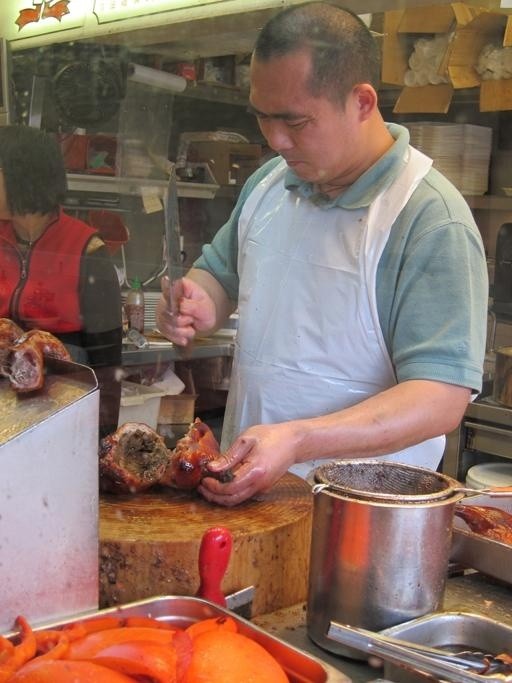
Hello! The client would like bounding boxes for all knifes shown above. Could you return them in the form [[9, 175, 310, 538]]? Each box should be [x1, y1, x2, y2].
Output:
[[163, 164, 194, 360]]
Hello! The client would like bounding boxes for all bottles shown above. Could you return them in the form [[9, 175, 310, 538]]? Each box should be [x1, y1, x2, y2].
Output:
[[121, 139, 160, 177], [127, 276, 144, 335]]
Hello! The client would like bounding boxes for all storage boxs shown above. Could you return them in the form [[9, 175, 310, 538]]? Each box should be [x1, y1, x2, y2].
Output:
[[378, 0, 512, 116]]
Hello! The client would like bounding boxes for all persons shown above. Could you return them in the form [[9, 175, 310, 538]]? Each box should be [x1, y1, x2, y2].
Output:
[[154, 2, 489, 509], [0, 123, 123, 444]]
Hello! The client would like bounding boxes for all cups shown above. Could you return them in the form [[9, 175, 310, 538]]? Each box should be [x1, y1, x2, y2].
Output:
[[306, 465, 467, 664], [403, 34, 450, 86]]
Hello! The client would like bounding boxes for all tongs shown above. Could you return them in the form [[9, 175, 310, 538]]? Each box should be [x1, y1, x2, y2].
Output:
[[323, 614, 511, 682]]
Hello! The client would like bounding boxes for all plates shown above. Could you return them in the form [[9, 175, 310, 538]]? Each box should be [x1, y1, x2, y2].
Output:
[[121, 292, 163, 329], [400, 120, 493, 196], [500, 186, 511, 197]]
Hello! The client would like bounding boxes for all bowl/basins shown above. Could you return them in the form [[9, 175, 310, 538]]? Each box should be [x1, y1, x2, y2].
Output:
[[176, 166, 205, 181]]
